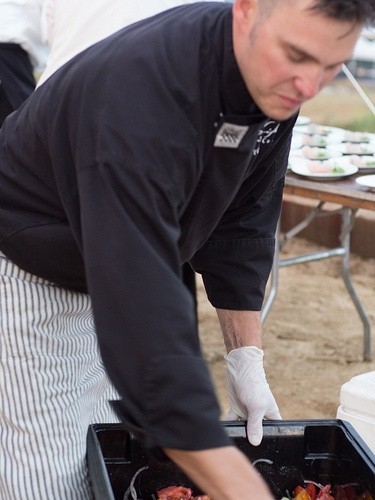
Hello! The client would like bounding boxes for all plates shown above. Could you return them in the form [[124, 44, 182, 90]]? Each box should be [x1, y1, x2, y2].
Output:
[[286, 114, 375, 194]]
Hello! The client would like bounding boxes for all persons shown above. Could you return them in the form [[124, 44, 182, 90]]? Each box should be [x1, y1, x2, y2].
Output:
[[0, 0, 375, 500], [0, 0, 53, 121]]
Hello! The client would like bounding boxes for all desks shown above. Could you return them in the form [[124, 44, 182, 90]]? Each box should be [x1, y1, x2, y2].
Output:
[[261, 171, 375, 363]]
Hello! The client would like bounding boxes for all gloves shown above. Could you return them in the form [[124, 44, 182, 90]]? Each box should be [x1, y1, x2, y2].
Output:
[[222, 346, 282, 446]]
